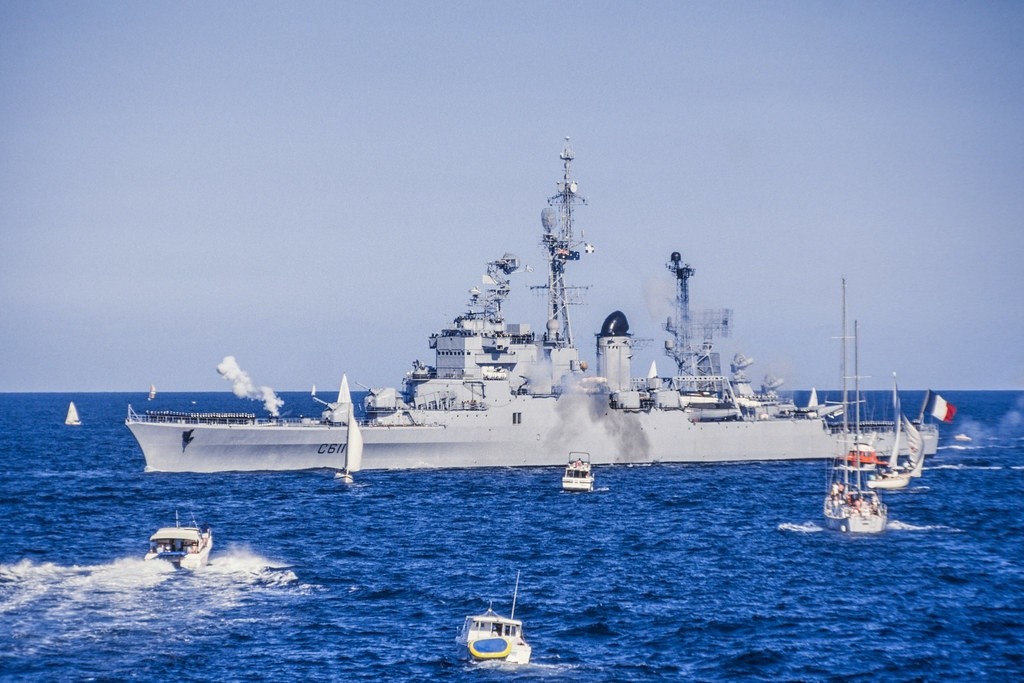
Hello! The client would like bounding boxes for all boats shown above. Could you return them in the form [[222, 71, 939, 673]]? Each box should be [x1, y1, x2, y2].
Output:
[[144, 505, 215, 571], [451, 569, 531, 669], [561, 451, 595, 492]]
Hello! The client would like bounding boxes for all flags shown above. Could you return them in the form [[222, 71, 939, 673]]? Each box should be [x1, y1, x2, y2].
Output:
[[557, 248, 580, 261], [929, 394, 959, 424], [584, 243, 594, 253]]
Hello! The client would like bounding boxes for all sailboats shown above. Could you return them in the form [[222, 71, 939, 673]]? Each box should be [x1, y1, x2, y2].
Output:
[[824, 276, 889, 535], [64, 402, 82, 426], [147, 384, 156, 402], [864, 378, 925, 491]]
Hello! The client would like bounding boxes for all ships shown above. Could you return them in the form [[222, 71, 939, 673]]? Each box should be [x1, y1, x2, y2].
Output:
[[125, 133, 957, 474]]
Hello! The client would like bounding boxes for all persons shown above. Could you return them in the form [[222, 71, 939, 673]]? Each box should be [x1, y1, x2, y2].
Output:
[[144, 410, 273, 425], [877, 468, 901, 480], [509, 329, 548, 344], [828, 483, 885, 517], [579, 458, 584, 466], [158, 541, 198, 554]]
